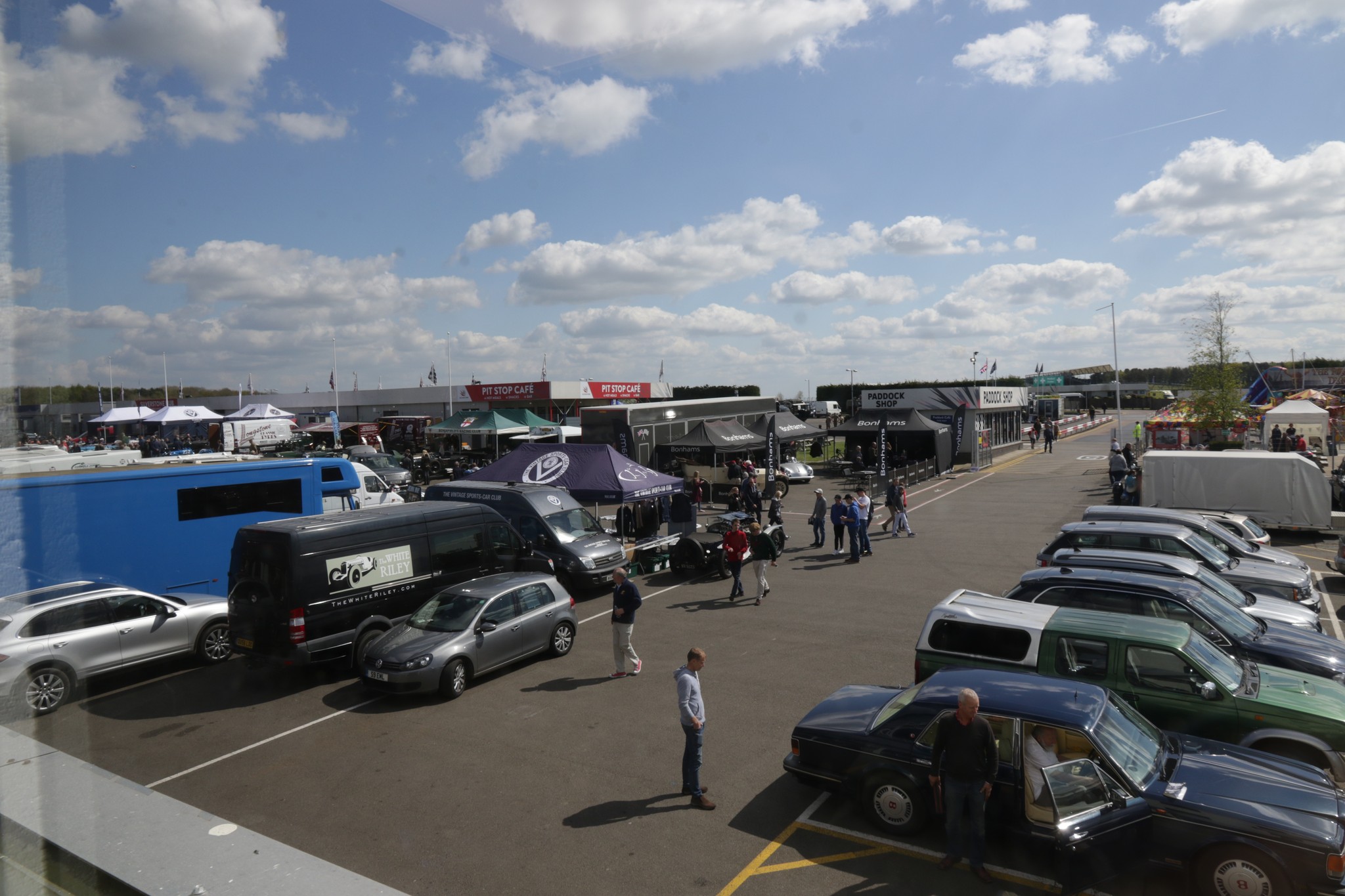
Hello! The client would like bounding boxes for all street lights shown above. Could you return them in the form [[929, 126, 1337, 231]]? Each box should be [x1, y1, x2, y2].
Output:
[[846, 368, 858, 417], [805, 379, 810, 403]]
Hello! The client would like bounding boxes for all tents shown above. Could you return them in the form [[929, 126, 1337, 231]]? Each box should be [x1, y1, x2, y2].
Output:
[[87, 404, 296, 439], [655, 407, 953, 511], [424, 409, 560, 462], [1263, 399, 1330, 455]]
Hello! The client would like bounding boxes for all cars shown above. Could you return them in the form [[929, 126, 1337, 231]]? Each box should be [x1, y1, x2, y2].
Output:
[[72, 427, 114, 443], [805, 401, 816, 419], [999, 504, 1345, 687], [26, 433, 40, 440], [1330, 455, 1345, 508], [781, 665, 1345, 895], [359, 570, 578, 701], [0, 580, 233, 718]]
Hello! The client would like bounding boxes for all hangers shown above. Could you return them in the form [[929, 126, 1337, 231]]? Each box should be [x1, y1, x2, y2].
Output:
[[624, 503, 626, 506]]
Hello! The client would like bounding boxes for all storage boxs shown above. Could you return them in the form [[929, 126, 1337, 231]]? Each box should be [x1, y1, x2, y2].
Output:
[[624, 543, 670, 578]]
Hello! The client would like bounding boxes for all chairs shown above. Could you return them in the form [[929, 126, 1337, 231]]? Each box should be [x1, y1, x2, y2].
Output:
[[1001, 719, 1078, 824], [1151, 539, 1160, 549], [1127, 648, 1192, 692], [1144, 599, 1168, 618], [827, 456, 892, 493]]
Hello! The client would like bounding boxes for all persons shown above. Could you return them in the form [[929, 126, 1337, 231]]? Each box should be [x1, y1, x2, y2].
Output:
[[608, 567, 642, 677], [674, 647, 717, 809], [0, 429, 512, 486], [1134, 421, 1141, 445], [1271, 423, 1306, 452], [1020, 403, 1108, 455], [614, 440, 917, 607], [925, 687, 1000, 884], [1024, 725, 1113, 808], [1109, 438, 1142, 506]]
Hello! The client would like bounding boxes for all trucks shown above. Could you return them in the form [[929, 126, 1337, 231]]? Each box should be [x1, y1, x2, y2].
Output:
[[210, 418, 300, 451]]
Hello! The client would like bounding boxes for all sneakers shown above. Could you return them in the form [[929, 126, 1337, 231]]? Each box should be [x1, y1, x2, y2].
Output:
[[633, 659, 642, 673], [784, 535, 791, 540], [608, 671, 626, 678], [810, 542, 823, 548], [831, 544, 873, 563], [881, 524, 920, 538]]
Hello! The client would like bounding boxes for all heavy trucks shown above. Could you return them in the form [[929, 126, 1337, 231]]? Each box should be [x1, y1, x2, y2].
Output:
[[0, 457, 359, 595]]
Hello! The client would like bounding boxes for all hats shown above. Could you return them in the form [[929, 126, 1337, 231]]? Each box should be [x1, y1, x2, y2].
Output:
[[843, 494, 852, 499], [834, 495, 841, 500], [322, 441, 327, 444], [855, 487, 864, 492], [749, 522, 760, 530], [814, 489, 823, 494], [732, 460, 736, 463]]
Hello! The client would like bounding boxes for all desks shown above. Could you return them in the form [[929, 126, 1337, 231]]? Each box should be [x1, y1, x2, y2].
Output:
[[836, 461, 852, 469], [852, 471, 876, 491], [635, 536, 681, 576], [1311, 454, 1326, 473], [601, 515, 616, 529]]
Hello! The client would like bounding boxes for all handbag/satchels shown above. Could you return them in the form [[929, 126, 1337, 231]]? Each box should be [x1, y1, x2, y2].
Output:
[[700, 492, 703, 497], [774, 511, 784, 524], [885, 501, 888, 506], [750, 466, 759, 477]]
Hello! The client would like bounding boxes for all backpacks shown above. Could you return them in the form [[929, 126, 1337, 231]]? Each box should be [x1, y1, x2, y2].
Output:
[[422, 457, 430, 470]]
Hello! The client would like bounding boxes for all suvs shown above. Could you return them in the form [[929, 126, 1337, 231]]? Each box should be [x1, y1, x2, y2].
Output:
[[913, 587, 1345, 784], [274, 432, 314, 452], [353, 452, 412, 491]]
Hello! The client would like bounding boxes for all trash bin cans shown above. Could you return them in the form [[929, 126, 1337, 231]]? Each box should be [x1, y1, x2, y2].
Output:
[[654, 553, 670, 569], [627, 562, 638, 578], [641, 557, 663, 572]]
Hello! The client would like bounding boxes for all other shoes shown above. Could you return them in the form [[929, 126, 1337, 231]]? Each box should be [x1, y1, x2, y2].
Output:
[[755, 600, 761, 605], [699, 510, 705, 512], [735, 593, 744, 597], [762, 589, 770, 597], [976, 867, 992, 881], [691, 794, 716, 809], [729, 596, 734, 601], [939, 855, 955, 870], [682, 786, 708, 794]]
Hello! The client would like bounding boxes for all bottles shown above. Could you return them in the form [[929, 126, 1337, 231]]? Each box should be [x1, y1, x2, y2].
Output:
[[614, 605, 621, 618], [770, 562, 778, 567]]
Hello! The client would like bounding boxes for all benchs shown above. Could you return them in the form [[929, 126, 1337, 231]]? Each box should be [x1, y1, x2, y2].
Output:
[[1319, 456, 1329, 467]]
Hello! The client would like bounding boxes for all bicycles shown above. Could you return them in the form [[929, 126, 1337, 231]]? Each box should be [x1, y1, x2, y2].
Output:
[[829, 448, 845, 467]]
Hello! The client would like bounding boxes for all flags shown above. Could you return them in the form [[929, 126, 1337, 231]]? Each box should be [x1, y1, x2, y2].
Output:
[[178, 382, 183, 398], [248, 374, 255, 396], [119, 384, 124, 401], [542, 358, 546, 382], [304, 364, 475, 393], [979, 361, 1043, 376]]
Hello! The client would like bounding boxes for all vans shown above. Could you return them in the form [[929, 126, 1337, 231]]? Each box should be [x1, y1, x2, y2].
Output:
[[322, 460, 405, 514], [1150, 390, 1175, 399], [227, 499, 556, 679], [424, 479, 632, 591], [1058, 393, 1085, 408], [816, 401, 842, 418]]
